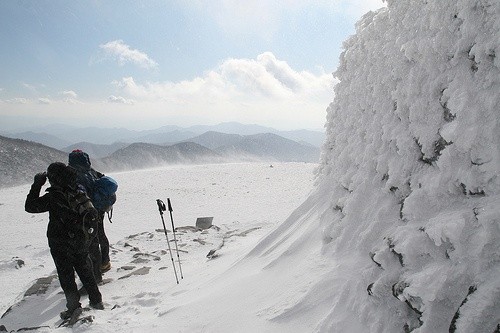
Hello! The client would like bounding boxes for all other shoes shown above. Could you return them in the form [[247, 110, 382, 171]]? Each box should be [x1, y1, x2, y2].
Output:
[[79, 285, 89, 296], [59, 308, 81, 320], [93, 274, 101, 283], [99, 262, 111, 273], [88, 302, 106, 310]]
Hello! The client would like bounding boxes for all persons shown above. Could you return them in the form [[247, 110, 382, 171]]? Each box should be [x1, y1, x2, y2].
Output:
[[68, 149, 111, 294], [25, 162, 104, 321]]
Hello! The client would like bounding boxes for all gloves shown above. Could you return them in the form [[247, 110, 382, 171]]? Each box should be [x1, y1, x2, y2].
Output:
[[34, 171, 48, 186]]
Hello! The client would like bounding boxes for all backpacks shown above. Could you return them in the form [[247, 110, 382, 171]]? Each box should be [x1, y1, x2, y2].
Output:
[[50, 185, 99, 250], [86, 169, 118, 224]]
